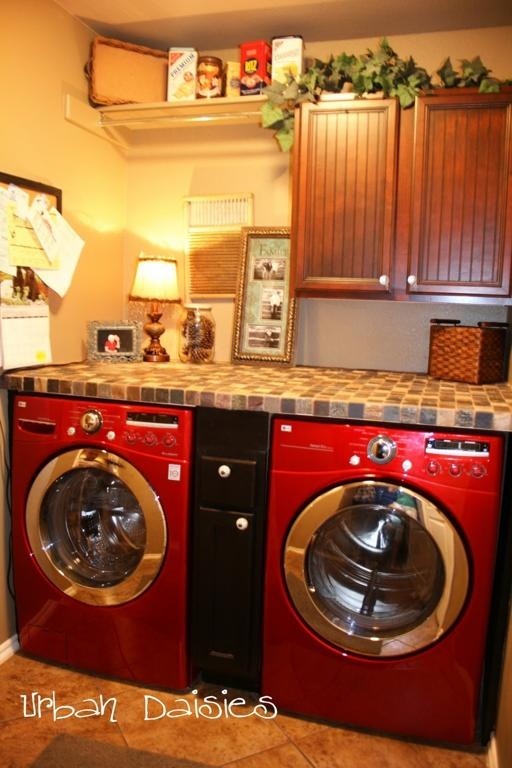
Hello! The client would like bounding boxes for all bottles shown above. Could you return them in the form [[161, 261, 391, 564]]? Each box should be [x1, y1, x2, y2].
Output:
[[177, 303, 216, 363]]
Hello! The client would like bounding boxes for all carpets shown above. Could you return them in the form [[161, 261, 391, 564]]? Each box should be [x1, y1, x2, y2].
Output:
[[29, 733, 216, 768]]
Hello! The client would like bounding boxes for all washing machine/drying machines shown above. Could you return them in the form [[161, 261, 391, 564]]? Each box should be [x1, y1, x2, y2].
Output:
[[262, 415, 505, 751], [8, 393, 197, 696]]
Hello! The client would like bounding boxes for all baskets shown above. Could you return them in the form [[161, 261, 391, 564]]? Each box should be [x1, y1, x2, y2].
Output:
[[428, 323, 506, 387]]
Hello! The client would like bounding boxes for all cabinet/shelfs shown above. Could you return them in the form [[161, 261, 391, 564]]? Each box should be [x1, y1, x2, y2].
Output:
[[288, 79, 512, 305]]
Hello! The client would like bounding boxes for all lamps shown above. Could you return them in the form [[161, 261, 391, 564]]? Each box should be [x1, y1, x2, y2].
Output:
[[128, 256, 181, 363]]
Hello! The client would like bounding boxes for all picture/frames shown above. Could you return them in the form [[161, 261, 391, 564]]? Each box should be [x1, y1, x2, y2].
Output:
[[231, 225, 298, 366], [87, 320, 143, 364]]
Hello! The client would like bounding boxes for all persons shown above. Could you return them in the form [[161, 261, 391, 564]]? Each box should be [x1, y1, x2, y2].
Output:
[[264, 330, 280, 346], [268, 260, 280, 279], [271, 291, 280, 318], [104, 333, 121, 353], [259, 260, 270, 278]]
